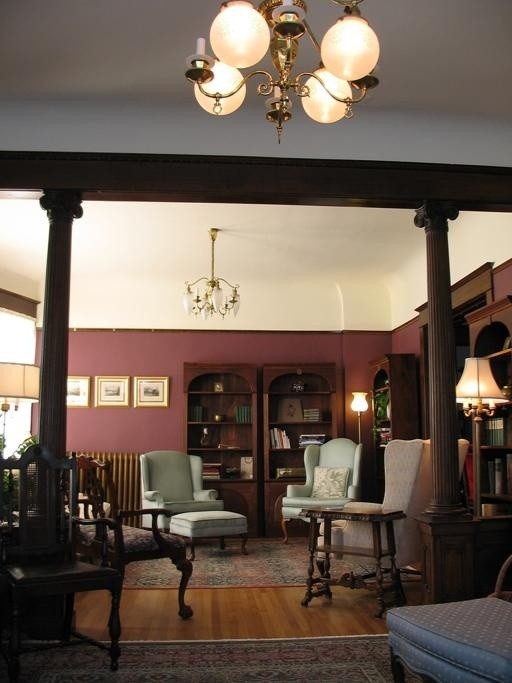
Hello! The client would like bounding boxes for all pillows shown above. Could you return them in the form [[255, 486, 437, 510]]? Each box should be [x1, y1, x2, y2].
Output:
[[311, 464, 350, 501]]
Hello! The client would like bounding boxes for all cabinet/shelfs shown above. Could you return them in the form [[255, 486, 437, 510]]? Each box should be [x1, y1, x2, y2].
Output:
[[461, 289, 511, 521], [180, 359, 261, 538], [254, 362, 340, 538], [366, 351, 422, 502]]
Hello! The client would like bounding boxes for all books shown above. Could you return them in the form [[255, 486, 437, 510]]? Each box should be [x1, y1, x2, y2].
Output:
[[189, 407, 203, 422], [235, 406, 252, 423], [303, 409, 322, 422], [485, 417, 512, 495], [270, 428, 290, 449], [203, 463, 223, 479]]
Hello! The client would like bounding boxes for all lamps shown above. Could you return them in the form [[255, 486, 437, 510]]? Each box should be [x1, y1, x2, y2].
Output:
[[451, 354, 508, 521], [349, 389, 369, 442], [183, 0, 387, 144], [0, 360, 41, 454], [183, 224, 243, 320]]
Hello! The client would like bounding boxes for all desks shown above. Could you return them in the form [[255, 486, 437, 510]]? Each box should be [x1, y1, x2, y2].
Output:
[[295, 503, 406, 624]]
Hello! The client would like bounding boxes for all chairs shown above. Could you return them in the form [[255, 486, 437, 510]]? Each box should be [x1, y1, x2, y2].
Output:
[[62, 449, 200, 625], [374, 543, 512, 683], [311, 435, 467, 583], [1, 443, 127, 683], [279, 434, 370, 543], [138, 445, 226, 556]]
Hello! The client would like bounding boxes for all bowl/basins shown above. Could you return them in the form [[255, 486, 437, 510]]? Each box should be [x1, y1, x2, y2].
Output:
[[213, 414, 226, 422]]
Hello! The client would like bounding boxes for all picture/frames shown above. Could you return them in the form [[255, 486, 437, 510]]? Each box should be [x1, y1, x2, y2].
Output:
[[131, 373, 170, 408], [66, 372, 93, 409], [93, 374, 132, 409]]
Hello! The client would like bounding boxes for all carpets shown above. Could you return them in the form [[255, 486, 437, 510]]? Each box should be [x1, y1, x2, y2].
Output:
[[76, 529, 423, 592], [0, 624, 435, 681]]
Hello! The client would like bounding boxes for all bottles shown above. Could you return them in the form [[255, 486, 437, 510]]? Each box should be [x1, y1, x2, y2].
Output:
[[501, 386, 512, 409], [200, 428, 210, 448]]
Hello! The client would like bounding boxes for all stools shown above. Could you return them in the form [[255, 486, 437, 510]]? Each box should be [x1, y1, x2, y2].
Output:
[[165, 508, 250, 560]]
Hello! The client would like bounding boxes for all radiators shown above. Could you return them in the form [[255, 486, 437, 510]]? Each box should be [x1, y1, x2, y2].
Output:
[[62, 446, 146, 530]]
